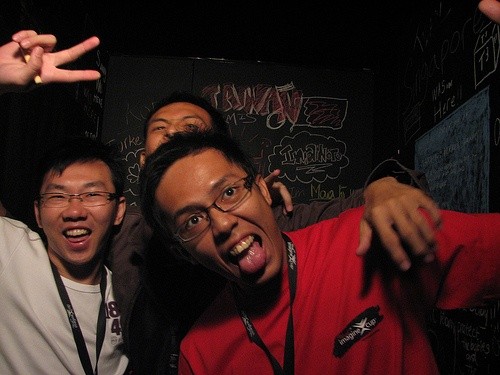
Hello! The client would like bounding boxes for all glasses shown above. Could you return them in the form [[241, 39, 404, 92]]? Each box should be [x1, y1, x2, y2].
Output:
[[170, 172, 257, 243], [37, 190, 120, 208]]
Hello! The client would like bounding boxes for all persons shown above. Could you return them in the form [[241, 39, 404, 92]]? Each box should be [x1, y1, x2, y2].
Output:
[[104, 91, 443, 375], [137, 1, 500, 375], [0, 30, 129, 375]]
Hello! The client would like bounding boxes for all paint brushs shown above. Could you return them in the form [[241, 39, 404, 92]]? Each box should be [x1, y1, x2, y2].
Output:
[[17, 39, 42, 85]]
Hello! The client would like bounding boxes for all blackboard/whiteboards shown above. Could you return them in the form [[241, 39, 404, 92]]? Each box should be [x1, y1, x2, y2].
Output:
[[78, 1, 500, 375]]
